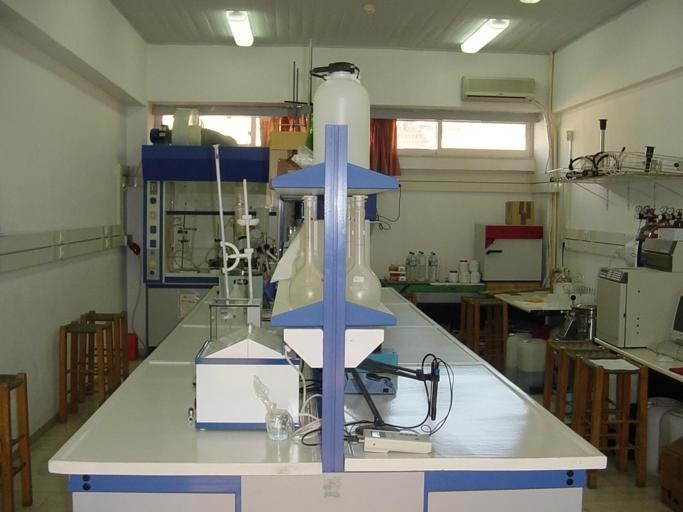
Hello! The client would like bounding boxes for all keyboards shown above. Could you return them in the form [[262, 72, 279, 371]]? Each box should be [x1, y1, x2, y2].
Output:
[[647, 340, 683, 361]]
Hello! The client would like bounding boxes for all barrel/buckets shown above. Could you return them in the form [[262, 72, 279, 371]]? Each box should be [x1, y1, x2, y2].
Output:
[[660, 408, 683, 469], [517, 339, 547, 388], [570, 304, 597, 340], [635, 397, 681, 475], [505, 333, 532, 383]]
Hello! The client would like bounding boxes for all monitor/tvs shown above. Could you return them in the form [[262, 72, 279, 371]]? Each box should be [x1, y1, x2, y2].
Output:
[[671, 295, 683, 340]]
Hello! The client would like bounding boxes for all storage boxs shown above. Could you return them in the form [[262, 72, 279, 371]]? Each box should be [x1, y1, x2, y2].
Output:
[[657, 432, 682, 512]]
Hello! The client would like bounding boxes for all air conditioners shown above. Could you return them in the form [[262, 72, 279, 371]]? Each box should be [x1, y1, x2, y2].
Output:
[[458, 74, 538, 105]]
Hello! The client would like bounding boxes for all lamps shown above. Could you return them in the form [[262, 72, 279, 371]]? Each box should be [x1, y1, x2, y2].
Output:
[[454, 15, 513, 56], [221, 6, 255, 51]]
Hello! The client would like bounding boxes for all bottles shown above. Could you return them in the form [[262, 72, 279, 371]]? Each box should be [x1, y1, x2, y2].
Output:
[[265, 408, 294, 440], [289, 195, 381, 309], [235, 187, 246, 248], [549, 266, 596, 306], [406, 250, 482, 283], [230, 284, 241, 309]]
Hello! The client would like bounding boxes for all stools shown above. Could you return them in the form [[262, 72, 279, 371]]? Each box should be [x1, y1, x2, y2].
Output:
[[457, 292, 505, 367], [562, 347, 621, 470], [79, 305, 130, 401], [572, 356, 649, 491], [54, 320, 117, 423], [542, 333, 607, 425], [0, 368, 38, 512]]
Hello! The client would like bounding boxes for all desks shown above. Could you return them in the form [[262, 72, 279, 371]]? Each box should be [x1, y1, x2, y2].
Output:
[[48, 268, 611, 512], [378, 280, 486, 306], [493, 291, 595, 316], [592, 334, 682, 391]]
